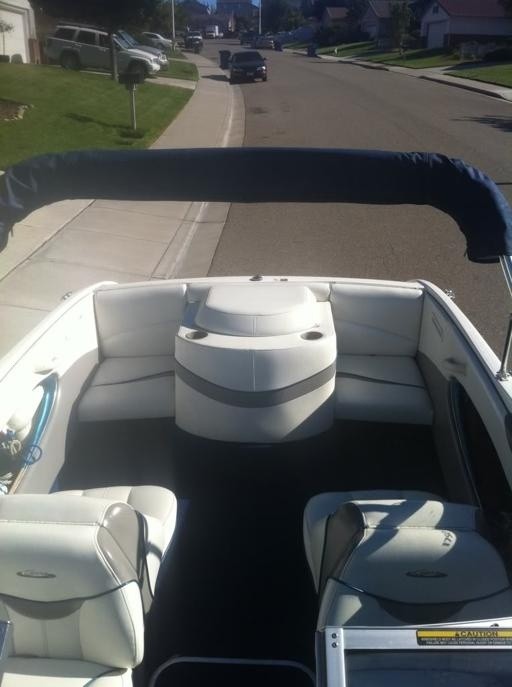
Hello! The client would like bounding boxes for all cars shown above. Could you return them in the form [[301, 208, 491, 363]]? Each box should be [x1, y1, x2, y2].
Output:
[[43, 22, 174, 79], [228, 51, 268, 82], [186, 31, 202, 46], [241, 32, 273, 49]]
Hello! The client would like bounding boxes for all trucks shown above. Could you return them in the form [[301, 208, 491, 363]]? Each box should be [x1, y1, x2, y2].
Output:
[[206, 25, 224, 39]]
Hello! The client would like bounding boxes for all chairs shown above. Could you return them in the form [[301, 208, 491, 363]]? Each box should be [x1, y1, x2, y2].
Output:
[[330, 284, 436, 438], [303, 491, 511, 626], [1, 483, 183, 687], [74, 283, 186, 439]]
